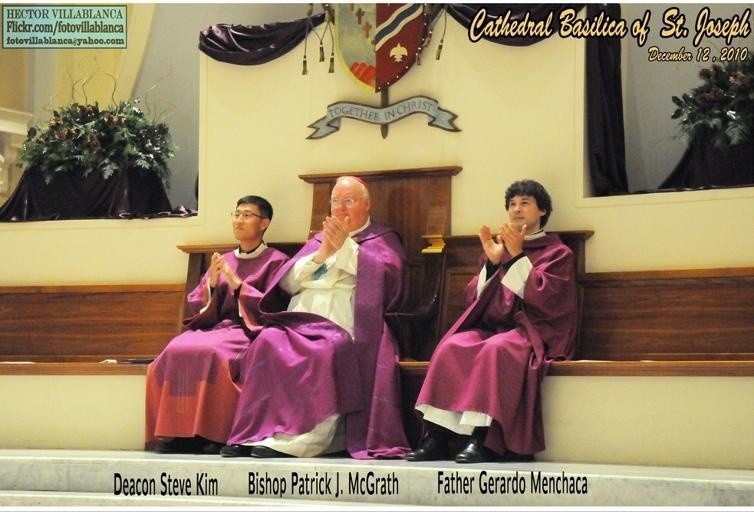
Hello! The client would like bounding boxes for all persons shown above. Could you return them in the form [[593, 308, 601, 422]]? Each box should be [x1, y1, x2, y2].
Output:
[[216, 174, 413, 460], [405, 179, 585, 464], [145, 194, 291, 454]]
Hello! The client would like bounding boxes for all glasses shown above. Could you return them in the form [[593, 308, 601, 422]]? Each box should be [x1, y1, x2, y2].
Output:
[[329, 197, 354, 209], [230, 210, 266, 219]]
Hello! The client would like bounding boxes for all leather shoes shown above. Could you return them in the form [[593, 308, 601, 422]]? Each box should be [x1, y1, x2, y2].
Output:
[[407, 434, 444, 460], [455, 438, 490, 463], [221, 443, 286, 455]]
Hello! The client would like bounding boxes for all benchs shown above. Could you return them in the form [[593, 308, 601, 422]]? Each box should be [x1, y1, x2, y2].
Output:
[[0, 167, 754, 377]]
